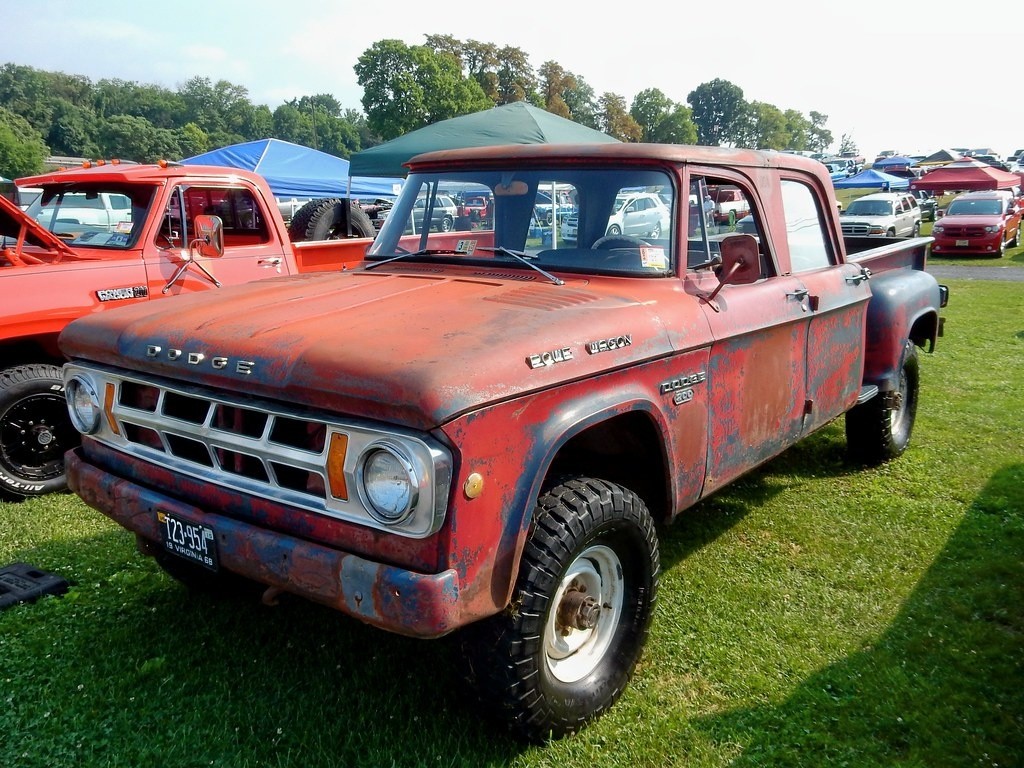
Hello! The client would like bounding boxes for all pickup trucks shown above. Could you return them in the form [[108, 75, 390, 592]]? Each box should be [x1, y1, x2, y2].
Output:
[[0, 159, 495, 500], [58, 142, 948, 740]]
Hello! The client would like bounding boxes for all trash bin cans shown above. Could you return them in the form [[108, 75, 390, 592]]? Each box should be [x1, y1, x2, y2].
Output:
[[542, 229, 552, 246]]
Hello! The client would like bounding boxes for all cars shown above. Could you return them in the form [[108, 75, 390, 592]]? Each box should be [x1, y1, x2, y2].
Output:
[[17, 188, 134, 234], [758, 148, 900, 177], [405, 192, 560, 233], [689, 189, 938, 238], [930, 191, 1021, 258], [561, 193, 670, 244]]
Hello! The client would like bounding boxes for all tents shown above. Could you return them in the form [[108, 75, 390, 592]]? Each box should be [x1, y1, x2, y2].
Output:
[[169, 139, 416, 248], [347, 102, 624, 250], [832, 149, 1022, 193]]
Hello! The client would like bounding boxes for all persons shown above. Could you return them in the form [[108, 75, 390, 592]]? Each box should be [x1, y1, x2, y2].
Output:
[[704, 195, 715, 227]]
